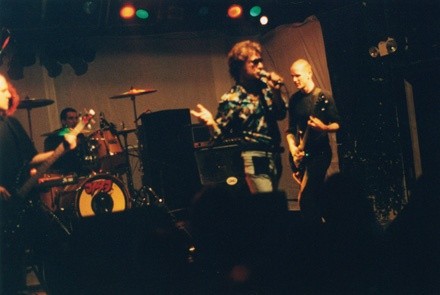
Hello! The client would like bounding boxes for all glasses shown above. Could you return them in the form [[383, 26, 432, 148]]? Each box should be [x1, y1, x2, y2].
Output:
[[251, 58, 263, 64]]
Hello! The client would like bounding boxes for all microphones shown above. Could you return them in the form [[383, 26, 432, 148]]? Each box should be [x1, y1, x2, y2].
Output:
[[259, 70, 285, 86], [100, 113, 104, 129]]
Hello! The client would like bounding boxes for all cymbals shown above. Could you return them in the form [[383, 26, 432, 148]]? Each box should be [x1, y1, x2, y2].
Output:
[[117, 127, 136, 135], [109, 86, 159, 100], [37, 127, 95, 138], [8, 96, 56, 110]]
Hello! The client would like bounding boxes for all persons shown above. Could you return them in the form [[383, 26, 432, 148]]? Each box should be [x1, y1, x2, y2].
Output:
[[189, 40, 288, 194], [0, 75, 78, 295], [285, 58, 345, 211], [44, 108, 100, 177]]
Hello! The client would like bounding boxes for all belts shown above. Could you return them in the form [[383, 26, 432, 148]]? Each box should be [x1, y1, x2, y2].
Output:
[[240, 145, 284, 153]]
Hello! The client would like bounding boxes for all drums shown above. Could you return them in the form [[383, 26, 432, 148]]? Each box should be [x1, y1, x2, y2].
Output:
[[55, 173, 131, 216], [34, 173, 62, 213], [85, 126, 128, 170]]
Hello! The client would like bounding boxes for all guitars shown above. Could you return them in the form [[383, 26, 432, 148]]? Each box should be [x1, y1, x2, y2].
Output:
[[288, 91, 330, 177], [1, 106, 97, 241]]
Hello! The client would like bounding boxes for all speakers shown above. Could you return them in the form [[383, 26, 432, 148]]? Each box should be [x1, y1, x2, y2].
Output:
[[140, 109, 238, 217]]
